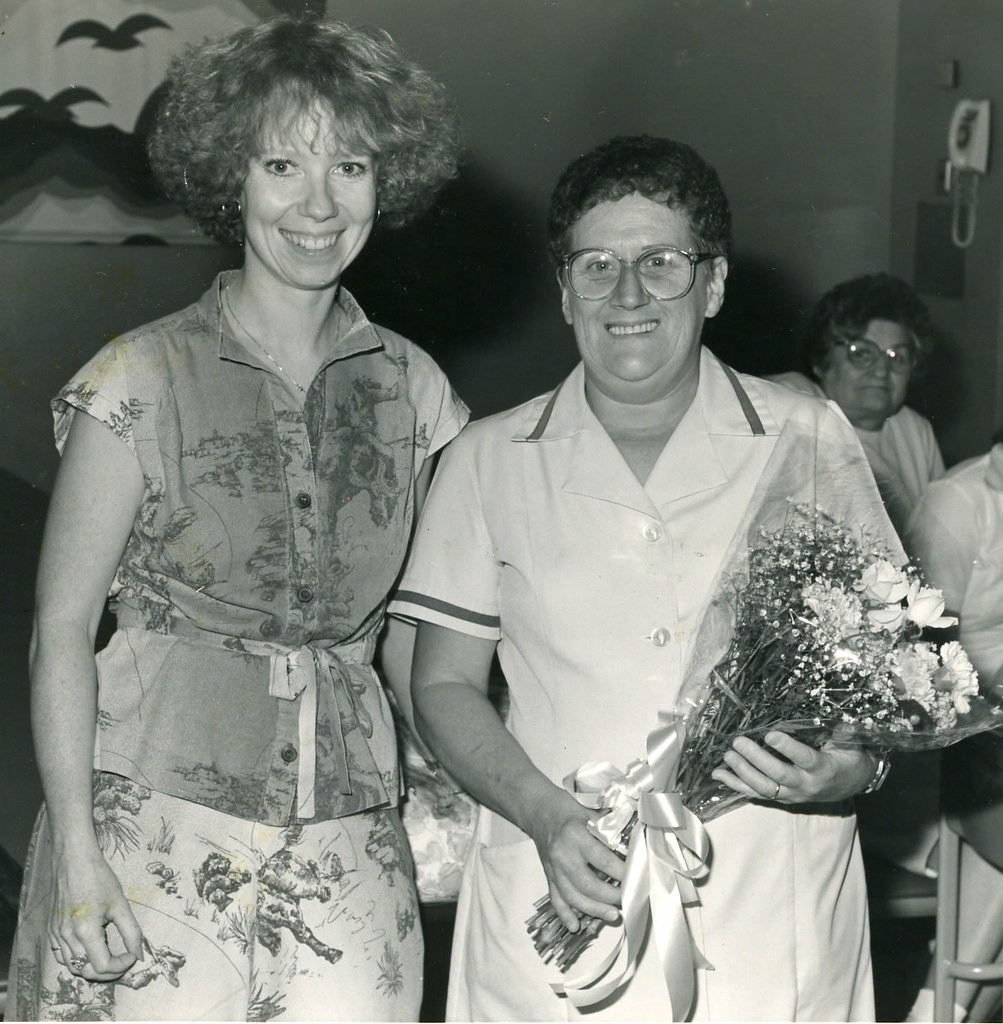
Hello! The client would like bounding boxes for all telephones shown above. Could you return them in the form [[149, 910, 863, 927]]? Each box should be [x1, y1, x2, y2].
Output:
[[948, 99, 991, 174]]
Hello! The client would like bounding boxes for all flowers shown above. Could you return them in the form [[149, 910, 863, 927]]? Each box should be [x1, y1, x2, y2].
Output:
[[528, 478, 1003, 969]]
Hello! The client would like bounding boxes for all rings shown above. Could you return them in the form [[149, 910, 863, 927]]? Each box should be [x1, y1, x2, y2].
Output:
[[50, 946, 61, 950], [70, 953, 89, 968], [769, 783, 779, 800]]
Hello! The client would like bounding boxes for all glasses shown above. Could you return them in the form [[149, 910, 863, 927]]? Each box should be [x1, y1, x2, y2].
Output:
[[829, 337, 921, 374], [558, 247, 719, 301]]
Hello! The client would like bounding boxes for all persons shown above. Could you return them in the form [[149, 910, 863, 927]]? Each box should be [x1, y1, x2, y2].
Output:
[[383, 130, 913, 1022], [3, 19, 471, 1024], [808, 271, 944, 553], [908, 422, 1001, 1020]]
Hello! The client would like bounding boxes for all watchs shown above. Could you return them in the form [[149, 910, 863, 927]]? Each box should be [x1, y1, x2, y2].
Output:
[[866, 760, 890, 794]]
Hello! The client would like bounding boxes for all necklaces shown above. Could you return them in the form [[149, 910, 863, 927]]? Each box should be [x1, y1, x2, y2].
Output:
[[223, 281, 342, 395]]
